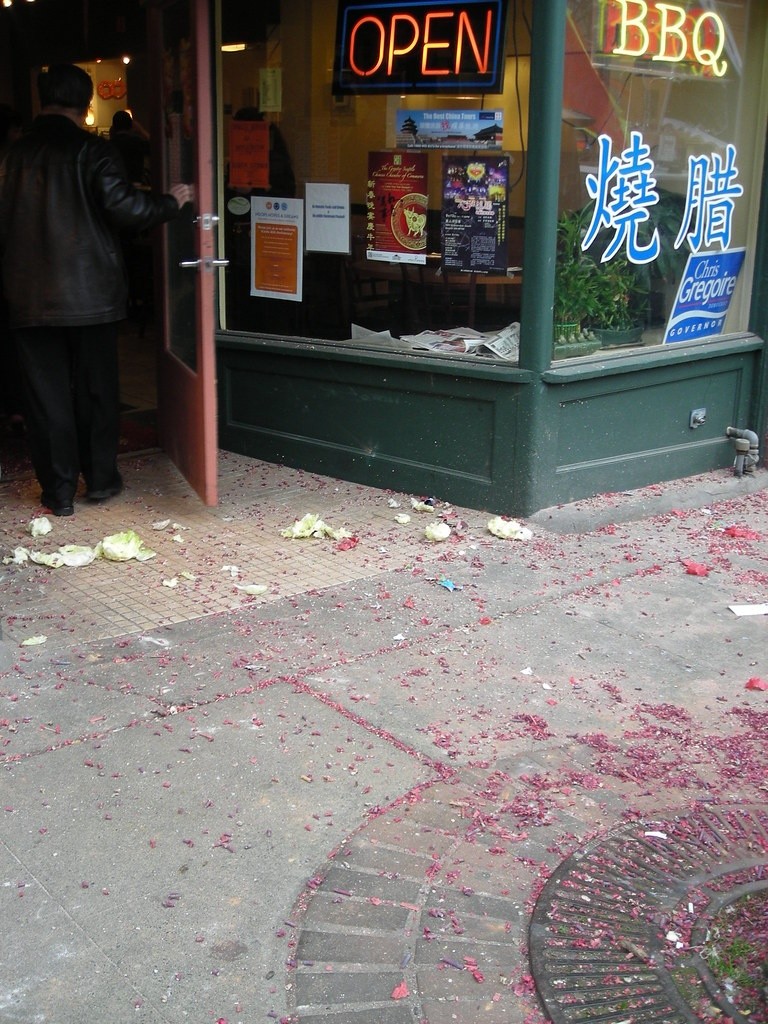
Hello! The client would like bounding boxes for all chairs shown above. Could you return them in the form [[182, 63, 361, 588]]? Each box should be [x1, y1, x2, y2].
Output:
[[400, 263, 481, 334]]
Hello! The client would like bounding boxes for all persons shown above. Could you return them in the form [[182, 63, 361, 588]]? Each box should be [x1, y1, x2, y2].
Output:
[[0, 60, 191, 516], [226, 106, 296, 222]]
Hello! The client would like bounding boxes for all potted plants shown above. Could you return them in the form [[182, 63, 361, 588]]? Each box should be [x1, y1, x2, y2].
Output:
[[555, 200, 650, 360]]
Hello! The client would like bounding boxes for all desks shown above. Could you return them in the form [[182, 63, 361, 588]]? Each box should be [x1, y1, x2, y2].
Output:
[[354, 261, 522, 319]]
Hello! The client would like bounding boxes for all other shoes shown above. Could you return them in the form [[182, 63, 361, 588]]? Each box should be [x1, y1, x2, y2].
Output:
[[87, 471, 123, 499], [41, 492, 74, 516], [6, 421, 31, 437]]
